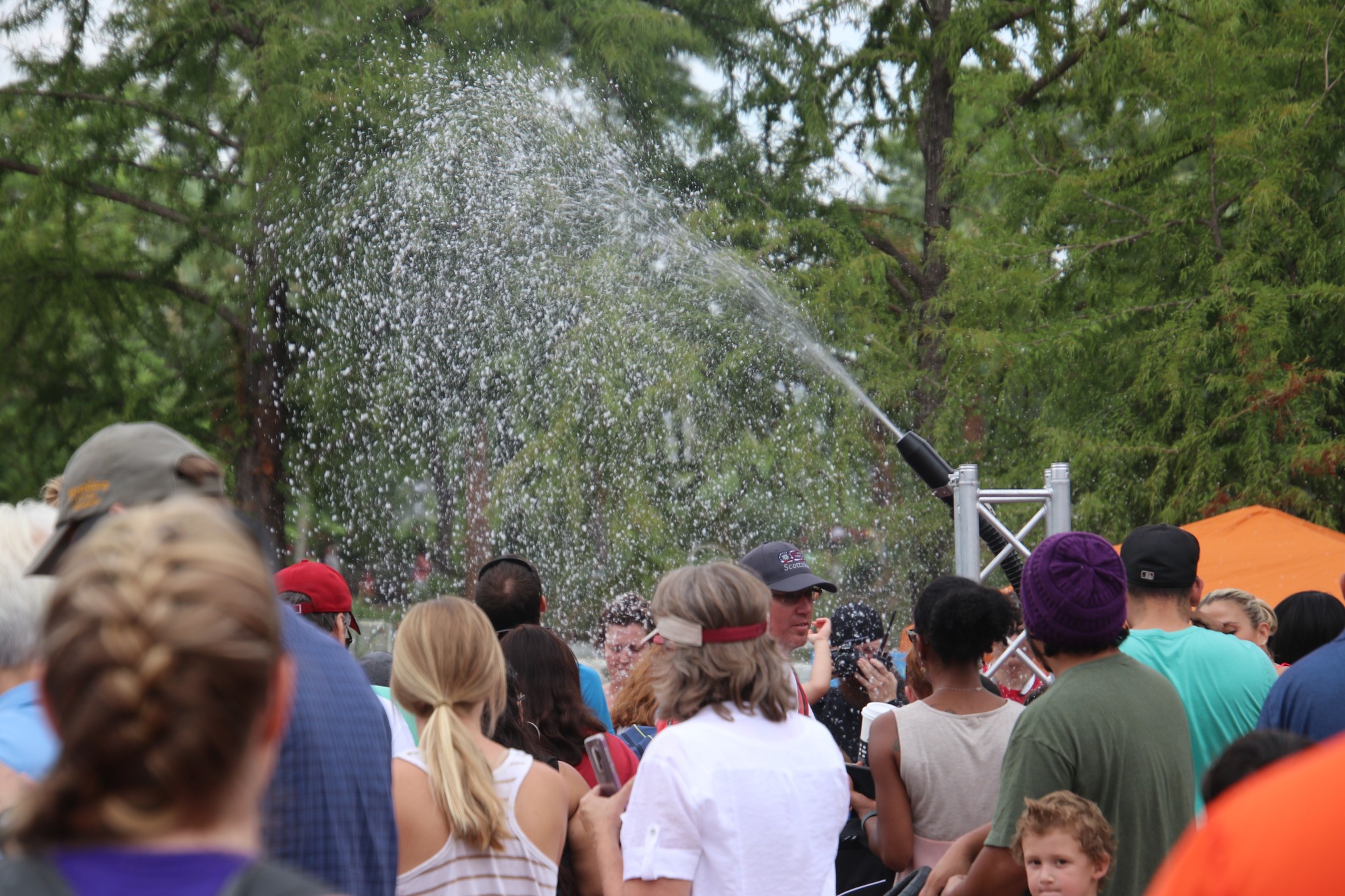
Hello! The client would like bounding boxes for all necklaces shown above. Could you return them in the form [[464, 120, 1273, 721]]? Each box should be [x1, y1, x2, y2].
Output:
[[931, 686, 986, 692]]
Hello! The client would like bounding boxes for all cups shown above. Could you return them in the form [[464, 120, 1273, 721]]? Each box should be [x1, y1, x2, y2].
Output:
[[858, 701, 898, 767]]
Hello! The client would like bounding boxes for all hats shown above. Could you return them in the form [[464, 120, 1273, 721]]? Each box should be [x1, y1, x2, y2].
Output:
[[1018, 530, 1129, 637], [831, 602, 886, 646], [735, 541, 838, 595], [266, 560, 365, 637], [1265, 590, 1345, 665], [20, 420, 225, 575], [1117, 521, 1201, 585]]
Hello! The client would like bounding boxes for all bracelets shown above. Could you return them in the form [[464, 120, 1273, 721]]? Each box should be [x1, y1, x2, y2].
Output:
[[860, 810, 878, 832], [887, 699, 899, 708]]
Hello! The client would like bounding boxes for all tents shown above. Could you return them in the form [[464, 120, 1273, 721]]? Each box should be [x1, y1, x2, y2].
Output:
[[899, 504, 1345, 654]]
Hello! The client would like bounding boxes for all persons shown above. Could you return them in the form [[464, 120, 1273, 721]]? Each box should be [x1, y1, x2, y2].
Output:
[[0, 423, 1345, 896]]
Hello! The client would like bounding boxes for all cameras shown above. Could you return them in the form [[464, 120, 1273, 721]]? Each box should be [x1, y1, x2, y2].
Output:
[[830, 645, 865, 677]]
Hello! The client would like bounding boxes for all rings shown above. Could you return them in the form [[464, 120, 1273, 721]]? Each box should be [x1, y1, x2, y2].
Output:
[[869, 676, 874, 682]]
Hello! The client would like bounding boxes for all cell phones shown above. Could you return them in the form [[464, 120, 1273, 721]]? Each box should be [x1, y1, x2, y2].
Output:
[[585, 736, 627, 811]]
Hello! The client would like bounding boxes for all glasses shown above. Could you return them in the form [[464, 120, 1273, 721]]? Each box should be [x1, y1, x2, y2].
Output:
[[771, 584, 823, 606]]
